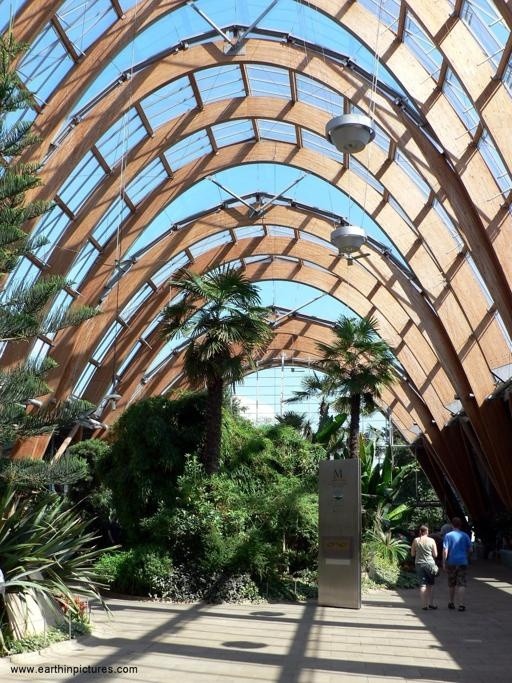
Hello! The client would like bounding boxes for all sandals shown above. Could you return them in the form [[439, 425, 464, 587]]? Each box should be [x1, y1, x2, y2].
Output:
[[448, 602, 455, 609], [458, 605, 466, 612]]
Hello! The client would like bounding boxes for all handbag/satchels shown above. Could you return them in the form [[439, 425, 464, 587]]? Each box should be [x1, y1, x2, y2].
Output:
[[431, 565, 439, 576]]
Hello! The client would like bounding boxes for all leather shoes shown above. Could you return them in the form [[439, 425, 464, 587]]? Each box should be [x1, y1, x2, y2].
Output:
[[422, 607, 428, 610], [428, 605, 437, 609]]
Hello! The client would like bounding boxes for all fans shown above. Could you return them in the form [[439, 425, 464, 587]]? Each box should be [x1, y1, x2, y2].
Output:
[[329, 215, 370, 266]]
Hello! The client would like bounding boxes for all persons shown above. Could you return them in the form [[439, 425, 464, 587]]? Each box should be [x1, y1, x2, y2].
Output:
[[441, 520, 452, 540], [411, 524, 439, 610], [443, 518, 473, 611]]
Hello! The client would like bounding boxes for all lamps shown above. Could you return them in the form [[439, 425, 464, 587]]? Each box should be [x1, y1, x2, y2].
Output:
[[104, 102, 126, 411], [316, 136, 367, 253], [298, 0, 385, 155]]
